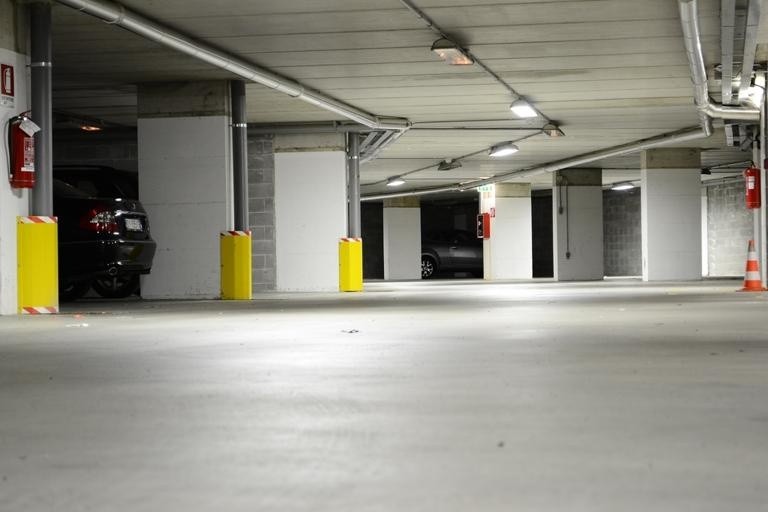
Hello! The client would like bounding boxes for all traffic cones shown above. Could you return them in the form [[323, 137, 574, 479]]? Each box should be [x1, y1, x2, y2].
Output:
[[736, 239, 768, 292]]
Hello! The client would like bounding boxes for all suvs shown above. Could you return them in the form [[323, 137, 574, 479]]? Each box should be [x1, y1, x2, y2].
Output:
[[53, 162, 157, 305]]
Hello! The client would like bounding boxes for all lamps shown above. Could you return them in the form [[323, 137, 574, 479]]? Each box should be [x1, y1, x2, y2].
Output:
[[385, 123, 564, 189], [431, 38, 539, 120]]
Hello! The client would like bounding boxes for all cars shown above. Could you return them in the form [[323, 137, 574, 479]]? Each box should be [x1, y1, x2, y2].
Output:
[[370, 227, 483, 279]]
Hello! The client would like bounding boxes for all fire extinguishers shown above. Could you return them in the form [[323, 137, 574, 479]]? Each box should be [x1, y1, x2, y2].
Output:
[[8, 110, 36, 188], [744, 160, 760, 208]]
